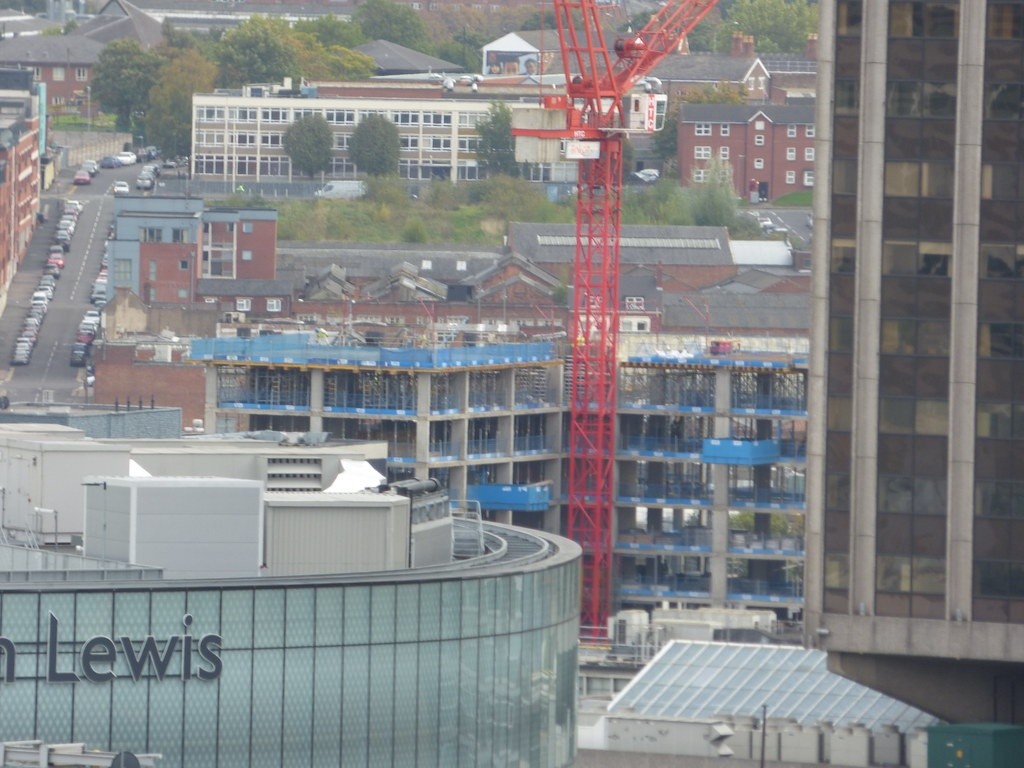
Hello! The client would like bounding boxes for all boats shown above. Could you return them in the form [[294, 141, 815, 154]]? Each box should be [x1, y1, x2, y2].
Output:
[[11, 201, 83, 366], [71, 219, 115, 369]]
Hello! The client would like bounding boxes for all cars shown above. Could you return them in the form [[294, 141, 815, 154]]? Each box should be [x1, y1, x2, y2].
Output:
[[163, 154, 188, 169], [743, 208, 813, 251], [134, 145, 162, 162], [73, 171, 91, 185], [136, 164, 160, 189], [114, 181, 130, 197], [81, 151, 137, 176]]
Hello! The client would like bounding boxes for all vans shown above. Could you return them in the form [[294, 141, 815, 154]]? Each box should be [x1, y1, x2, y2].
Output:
[[314, 180, 365, 199]]
